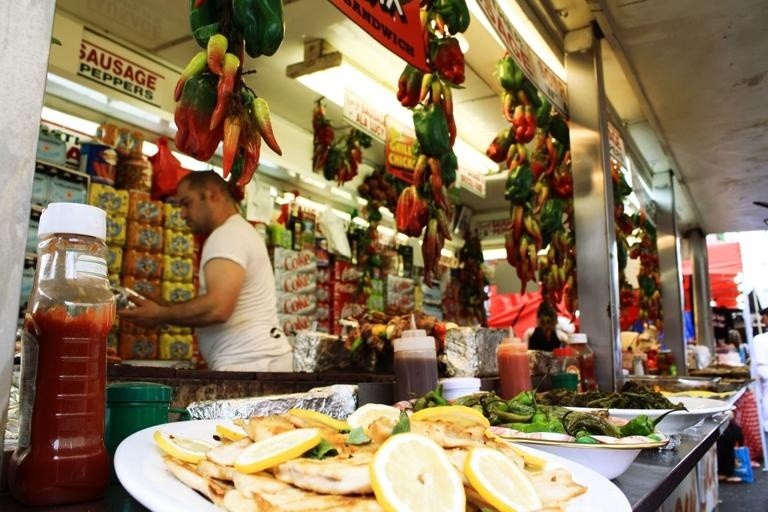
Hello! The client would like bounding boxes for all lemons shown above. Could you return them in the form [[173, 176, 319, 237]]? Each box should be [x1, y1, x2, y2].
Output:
[[153, 402, 587, 512]]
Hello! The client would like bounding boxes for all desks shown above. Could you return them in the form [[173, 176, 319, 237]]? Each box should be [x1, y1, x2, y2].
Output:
[[109, 404, 730, 512]]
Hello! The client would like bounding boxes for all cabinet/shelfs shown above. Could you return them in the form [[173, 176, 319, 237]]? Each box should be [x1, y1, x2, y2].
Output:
[[13, 124, 441, 382]]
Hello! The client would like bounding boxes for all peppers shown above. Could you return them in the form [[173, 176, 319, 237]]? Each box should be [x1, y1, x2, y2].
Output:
[[174, 0, 286, 186], [312, 0, 664, 336], [404, 366, 690, 443]]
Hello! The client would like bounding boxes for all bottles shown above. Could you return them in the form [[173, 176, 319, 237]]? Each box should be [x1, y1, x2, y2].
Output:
[[567, 333, 599, 393], [271, 201, 306, 252], [34, 124, 87, 187], [631, 344, 677, 377], [496, 325, 533, 399], [389, 314, 439, 408], [7, 201, 118, 509], [549, 345, 584, 394], [81, 118, 154, 196]]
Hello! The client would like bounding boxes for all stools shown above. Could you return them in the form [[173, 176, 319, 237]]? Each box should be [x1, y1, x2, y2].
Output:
[[734, 446, 755, 482]]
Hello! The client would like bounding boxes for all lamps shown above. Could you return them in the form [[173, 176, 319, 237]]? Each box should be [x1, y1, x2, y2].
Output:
[[284, 39, 501, 181], [46, 69, 287, 176]]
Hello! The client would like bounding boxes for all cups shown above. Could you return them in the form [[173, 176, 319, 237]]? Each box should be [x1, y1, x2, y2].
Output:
[[103, 379, 193, 458], [439, 376, 483, 403], [548, 369, 590, 398]]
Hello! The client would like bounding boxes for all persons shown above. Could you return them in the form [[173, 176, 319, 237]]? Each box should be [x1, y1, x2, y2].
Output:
[[528, 300, 561, 351], [116, 170, 294, 373], [713, 307, 768, 485], [655, 315, 666, 350]]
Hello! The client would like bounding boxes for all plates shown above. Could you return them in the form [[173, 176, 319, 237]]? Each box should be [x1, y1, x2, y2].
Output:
[[660, 367, 753, 399], [555, 390, 736, 449], [111, 415, 634, 512], [394, 393, 667, 480]]
